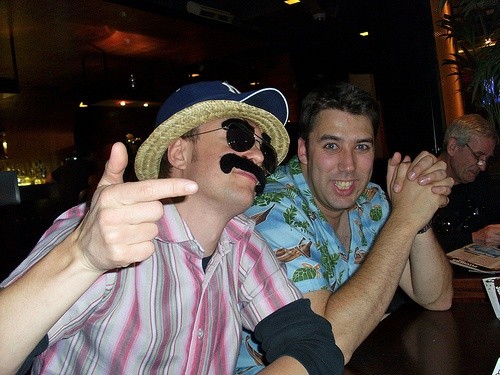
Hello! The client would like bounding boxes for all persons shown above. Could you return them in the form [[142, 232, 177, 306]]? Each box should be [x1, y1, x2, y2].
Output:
[[233, 81, 454, 375], [431, 113, 500, 254], [0, 81, 345, 375]]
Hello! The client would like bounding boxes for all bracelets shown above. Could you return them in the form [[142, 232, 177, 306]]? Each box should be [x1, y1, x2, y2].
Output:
[[416, 219, 432, 234]]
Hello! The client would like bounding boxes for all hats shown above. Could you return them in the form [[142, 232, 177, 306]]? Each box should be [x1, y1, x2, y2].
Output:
[[157, 80, 289, 126], [134, 98, 291, 181]]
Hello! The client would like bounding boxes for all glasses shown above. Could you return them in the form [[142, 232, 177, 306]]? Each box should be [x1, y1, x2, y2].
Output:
[[450, 137, 496, 165], [181, 122, 277, 177]]
